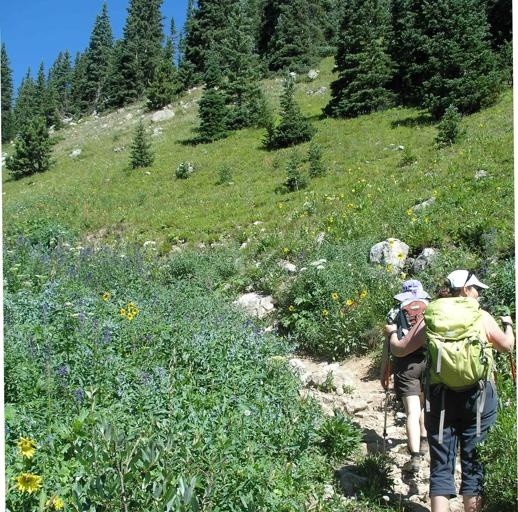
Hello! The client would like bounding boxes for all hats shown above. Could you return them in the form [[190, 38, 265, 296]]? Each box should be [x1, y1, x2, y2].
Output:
[[447, 268, 489, 291], [393, 280, 432, 302]]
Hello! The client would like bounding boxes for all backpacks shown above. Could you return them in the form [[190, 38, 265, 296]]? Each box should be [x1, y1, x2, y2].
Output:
[[397, 297, 428, 339], [424, 297, 493, 389]]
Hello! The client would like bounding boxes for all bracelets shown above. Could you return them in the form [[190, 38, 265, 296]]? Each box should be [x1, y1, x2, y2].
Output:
[[388, 329, 398, 337], [502, 321, 512, 327]]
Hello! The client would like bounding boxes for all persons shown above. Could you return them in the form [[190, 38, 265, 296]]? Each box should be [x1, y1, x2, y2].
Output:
[[384, 269, 517, 511], [380, 278, 433, 475]]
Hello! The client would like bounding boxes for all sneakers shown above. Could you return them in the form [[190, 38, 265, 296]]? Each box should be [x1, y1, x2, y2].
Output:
[[419, 437, 429, 455], [400, 453, 422, 473]]
